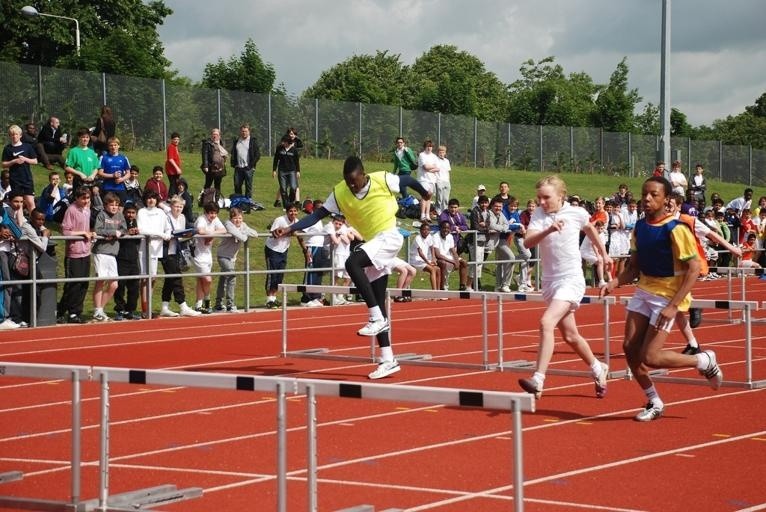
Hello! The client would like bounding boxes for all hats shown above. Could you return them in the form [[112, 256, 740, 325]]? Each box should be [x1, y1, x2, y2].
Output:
[[477, 184, 486, 191]]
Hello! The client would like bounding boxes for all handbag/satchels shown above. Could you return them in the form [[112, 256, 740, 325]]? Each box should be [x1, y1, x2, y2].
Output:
[[454, 236, 466, 254], [176, 248, 190, 274], [7, 248, 30, 278]]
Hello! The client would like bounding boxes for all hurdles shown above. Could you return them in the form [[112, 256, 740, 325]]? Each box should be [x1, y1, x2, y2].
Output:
[[618, 294, 766, 391], [1, 360, 178, 512], [278, 283, 417, 363], [496, 291, 630, 379], [389, 288, 529, 372], [294, 377, 538, 512], [89, 365, 297, 512], [693, 269, 766, 325]]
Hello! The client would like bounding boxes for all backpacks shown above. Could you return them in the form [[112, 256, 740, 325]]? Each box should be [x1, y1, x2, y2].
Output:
[[198, 187, 227, 209]]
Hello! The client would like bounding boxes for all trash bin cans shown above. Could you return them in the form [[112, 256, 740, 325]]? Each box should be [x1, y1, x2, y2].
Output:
[[34, 240, 58, 327]]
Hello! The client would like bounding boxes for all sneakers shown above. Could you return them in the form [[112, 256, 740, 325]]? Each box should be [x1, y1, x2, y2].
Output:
[[264, 294, 353, 306], [599, 280, 608, 288], [493, 274, 534, 292], [636, 400, 664, 423], [592, 360, 609, 398], [680, 344, 701, 356], [690, 307, 701, 328], [54, 302, 151, 325], [158, 299, 239, 318], [518, 376, 542, 399], [0, 317, 29, 330], [393, 294, 412, 302], [366, 359, 400, 380], [701, 349, 723, 391], [355, 318, 391, 336], [707, 271, 721, 281]]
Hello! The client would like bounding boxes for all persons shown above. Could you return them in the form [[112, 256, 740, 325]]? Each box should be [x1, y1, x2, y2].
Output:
[[389, 137, 765, 304], [517, 175, 613, 398], [263, 200, 366, 310], [600, 177, 723, 422], [1, 106, 261, 330], [273, 127, 304, 209], [668, 192, 742, 355], [272, 157, 432, 380]]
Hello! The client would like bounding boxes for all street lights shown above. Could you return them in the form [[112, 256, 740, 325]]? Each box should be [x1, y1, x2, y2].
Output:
[[21, 4, 82, 59]]
